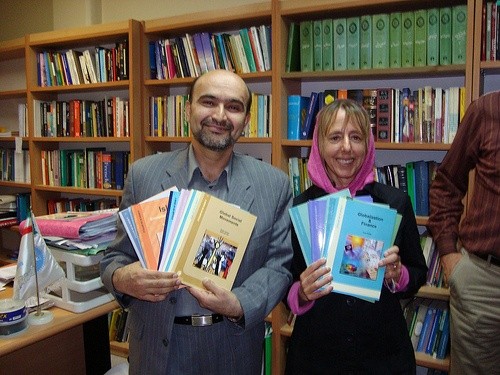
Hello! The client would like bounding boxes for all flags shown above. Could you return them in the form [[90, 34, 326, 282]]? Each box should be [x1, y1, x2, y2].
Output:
[[13, 209, 66, 305]]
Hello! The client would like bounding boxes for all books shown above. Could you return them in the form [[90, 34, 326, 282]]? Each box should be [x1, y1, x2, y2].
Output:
[[287, 4, 467, 72], [417, 230, 450, 290], [287, 147, 314, 198], [244, 92, 273, 138], [47, 198, 117, 215], [403, 295, 450, 360], [263, 324, 273, 375], [149, 93, 190, 138], [482, 0, 500, 63], [0, 141, 31, 183], [107, 307, 132, 345], [289, 188, 402, 303], [374, 161, 436, 218], [117, 186, 256, 295], [149, 25, 271, 80], [0, 193, 28, 229], [37, 39, 128, 87], [40, 146, 129, 192], [288, 86, 466, 145], [0, 102, 28, 137], [37, 93, 129, 138]]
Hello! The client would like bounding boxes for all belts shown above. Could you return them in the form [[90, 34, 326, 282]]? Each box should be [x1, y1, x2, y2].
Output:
[[173, 312, 223, 327], [471, 251, 500, 269]]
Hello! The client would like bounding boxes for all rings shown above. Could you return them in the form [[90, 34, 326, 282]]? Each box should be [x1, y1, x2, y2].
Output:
[[393, 265, 397, 271]]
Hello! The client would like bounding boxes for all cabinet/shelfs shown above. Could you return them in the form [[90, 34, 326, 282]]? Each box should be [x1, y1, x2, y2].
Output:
[[0, 0, 500, 375]]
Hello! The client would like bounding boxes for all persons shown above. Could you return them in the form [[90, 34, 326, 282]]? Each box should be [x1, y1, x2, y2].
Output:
[[279, 99, 428, 375], [94, 72, 297, 375], [429, 90, 500, 375]]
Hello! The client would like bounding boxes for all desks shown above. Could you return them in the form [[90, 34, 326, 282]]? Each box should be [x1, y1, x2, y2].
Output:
[[0, 262, 120, 375]]
